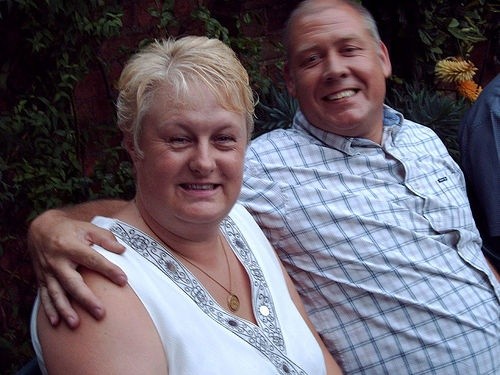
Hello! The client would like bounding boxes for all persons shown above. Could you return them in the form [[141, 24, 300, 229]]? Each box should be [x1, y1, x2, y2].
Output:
[[28, 0, 500, 375], [31, 36, 345, 375]]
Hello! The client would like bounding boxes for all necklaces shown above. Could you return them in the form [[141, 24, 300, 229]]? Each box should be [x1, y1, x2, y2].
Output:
[[146, 222, 241, 312]]
